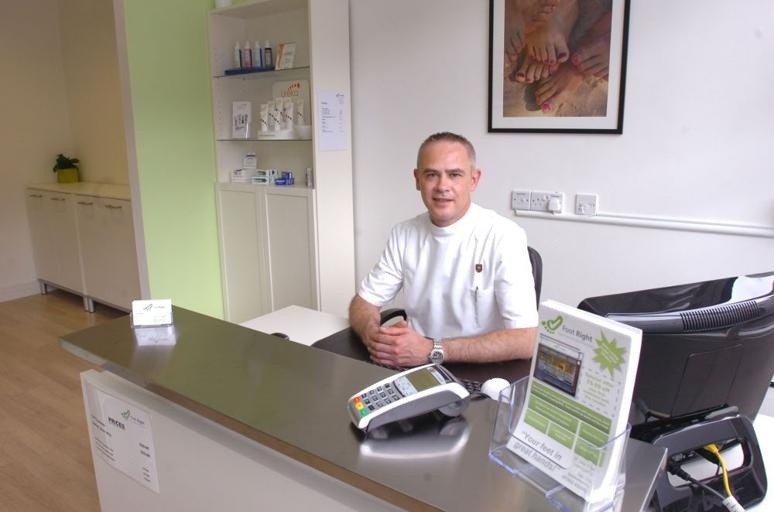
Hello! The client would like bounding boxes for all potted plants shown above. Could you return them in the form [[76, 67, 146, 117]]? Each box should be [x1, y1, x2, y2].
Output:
[[53, 152, 80, 182]]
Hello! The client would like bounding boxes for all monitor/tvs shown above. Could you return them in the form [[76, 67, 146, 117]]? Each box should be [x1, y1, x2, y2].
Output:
[[577, 271, 774, 511]]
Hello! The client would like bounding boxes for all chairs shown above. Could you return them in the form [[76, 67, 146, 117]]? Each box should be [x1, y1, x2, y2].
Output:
[[575, 270, 774, 454], [526, 248, 542, 308]]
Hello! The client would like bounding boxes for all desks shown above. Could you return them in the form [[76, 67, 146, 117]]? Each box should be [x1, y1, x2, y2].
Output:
[[58, 304, 672, 510]]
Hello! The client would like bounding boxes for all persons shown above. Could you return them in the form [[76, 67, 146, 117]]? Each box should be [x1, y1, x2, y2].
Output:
[[504, 0, 612, 115], [349, 131, 539, 368]]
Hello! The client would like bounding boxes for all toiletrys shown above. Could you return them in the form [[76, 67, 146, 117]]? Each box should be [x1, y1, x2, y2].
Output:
[[232, 38, 272, 69], [259, 97, 304, 134]]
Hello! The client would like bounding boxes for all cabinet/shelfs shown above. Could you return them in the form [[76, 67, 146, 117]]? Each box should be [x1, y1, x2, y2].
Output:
[[26, 190, 47, 293], [97, 197, 140, 315], [205, 1, 354, 187], [48, 191, 86, 313], [209, 185, 355, 324], [72, 194, 102, 315]]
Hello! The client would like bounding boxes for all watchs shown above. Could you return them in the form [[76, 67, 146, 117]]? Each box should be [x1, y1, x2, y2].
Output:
[[429, 337, 445, 364]]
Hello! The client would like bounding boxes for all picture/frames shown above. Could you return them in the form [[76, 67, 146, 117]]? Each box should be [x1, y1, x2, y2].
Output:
[[488, 0, 630, 135]]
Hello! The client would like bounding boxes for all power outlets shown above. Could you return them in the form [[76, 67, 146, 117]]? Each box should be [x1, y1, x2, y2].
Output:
[[574, 194, 596, 215], [530, 192, 563, 213], [510, 190, 529, 210]]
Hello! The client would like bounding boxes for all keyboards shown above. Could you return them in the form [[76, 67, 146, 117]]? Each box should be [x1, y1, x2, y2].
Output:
[[459, 377, 483, 394]]
